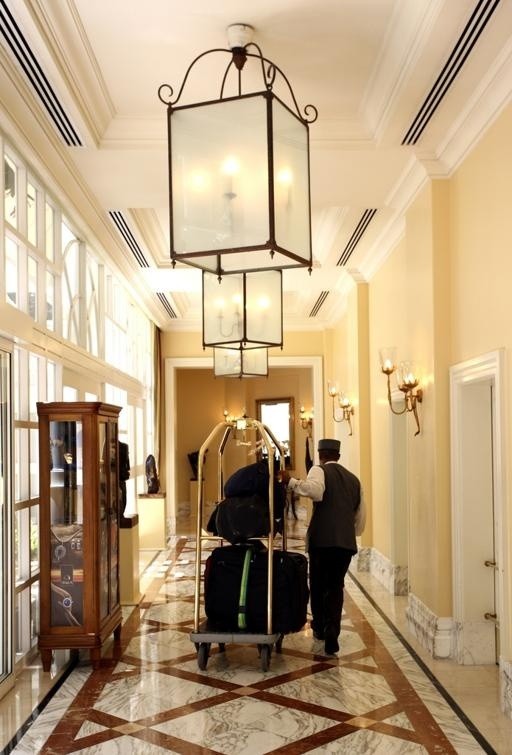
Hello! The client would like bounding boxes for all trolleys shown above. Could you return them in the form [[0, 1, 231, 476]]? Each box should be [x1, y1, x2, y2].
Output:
[[190, 415, 290, 673]]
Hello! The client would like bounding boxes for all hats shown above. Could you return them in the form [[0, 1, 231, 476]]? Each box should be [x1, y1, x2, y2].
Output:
[[317, 439, 341, 452]]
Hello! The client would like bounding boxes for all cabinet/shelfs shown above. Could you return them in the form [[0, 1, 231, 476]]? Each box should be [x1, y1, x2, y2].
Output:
[[37, 400, 126, 676]]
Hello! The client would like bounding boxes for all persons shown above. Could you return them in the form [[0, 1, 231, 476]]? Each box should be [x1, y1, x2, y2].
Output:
[[275, 439, 368, 656]]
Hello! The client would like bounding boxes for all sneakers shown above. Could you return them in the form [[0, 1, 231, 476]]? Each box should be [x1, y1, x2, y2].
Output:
[[312, 629, 324, 640], [324, 624, 339, 655]]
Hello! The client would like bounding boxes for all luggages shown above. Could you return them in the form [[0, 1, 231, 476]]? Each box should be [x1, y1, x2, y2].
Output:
[[206, 459, 284, 544], [203, 541, 310, 634]]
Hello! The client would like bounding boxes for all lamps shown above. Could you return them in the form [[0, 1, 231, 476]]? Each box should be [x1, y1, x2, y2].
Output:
[[378, 349, 423, 436], [213, 346, 269, 380], [326, 379, 354, 436], [296, 403, 313, 429], [155, 17, 320, 278], [202, 266, 283, 350]]
